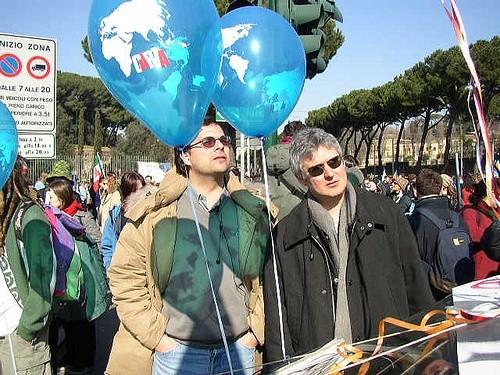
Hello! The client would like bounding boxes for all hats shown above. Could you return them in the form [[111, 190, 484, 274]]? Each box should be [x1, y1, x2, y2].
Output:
[[46, 160, 74, 185]]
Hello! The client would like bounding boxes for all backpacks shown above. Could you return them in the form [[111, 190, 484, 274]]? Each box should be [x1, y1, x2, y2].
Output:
[[411, 208, 472, 293]]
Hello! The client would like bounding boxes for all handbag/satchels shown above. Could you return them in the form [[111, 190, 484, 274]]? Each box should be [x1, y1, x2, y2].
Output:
[[43, 205, 107, 322]]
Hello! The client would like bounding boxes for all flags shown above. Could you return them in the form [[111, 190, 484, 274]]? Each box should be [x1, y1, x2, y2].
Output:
[[93, 154, 103, 191]]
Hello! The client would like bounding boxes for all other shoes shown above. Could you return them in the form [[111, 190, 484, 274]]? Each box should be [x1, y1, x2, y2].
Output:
[[56, 363, 94, 374]]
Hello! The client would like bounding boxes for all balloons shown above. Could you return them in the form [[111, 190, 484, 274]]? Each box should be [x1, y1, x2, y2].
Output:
[[0, 101, 18, 190], [87, 0, 223, 148], [201, 7, 306, 137]]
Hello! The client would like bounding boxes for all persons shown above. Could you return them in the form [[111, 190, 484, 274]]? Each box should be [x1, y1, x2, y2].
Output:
[[407, 170, 472, 301], [29, 189, 121, 375], [50, 181, 102, 375], [0, 155, 56, 375], [104, 115, 278, 374], [262, 129, 437, 375], [415, 348, 459, 375], [29, 122, 500, 281]]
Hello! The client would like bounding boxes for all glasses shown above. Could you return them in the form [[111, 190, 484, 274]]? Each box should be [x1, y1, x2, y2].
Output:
[[393, 181, 397, 184], [187, 135, 231, 148], [305, 155, 342, 177]]
